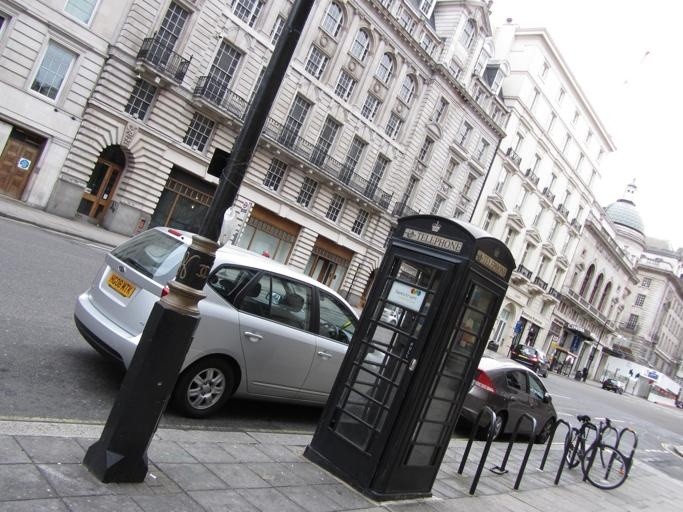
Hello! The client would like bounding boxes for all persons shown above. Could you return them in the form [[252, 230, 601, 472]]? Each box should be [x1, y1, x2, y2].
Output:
[[582, 365, 588, 382]]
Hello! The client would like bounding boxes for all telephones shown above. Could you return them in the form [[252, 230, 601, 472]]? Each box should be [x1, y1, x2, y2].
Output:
[[398, 310, 427, 347]]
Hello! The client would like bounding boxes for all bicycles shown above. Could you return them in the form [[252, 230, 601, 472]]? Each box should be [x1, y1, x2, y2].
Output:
[[565, 415, 630, 489]]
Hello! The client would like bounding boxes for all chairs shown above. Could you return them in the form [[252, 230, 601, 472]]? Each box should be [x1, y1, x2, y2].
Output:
[[239, 278, 305, 329]]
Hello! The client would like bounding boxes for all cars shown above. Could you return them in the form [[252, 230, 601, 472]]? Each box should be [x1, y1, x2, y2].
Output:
[[73, 227, 388, 420], [510, 345, 549, 379], [461, 345, 557, 444], [601, 378, 623, 394]]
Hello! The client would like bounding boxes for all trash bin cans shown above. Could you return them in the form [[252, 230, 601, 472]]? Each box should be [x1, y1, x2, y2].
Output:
[[488, 341, 500, 352]]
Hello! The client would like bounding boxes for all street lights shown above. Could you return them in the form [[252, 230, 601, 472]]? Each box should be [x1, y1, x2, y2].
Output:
[[583, 320, 624, 381]]
